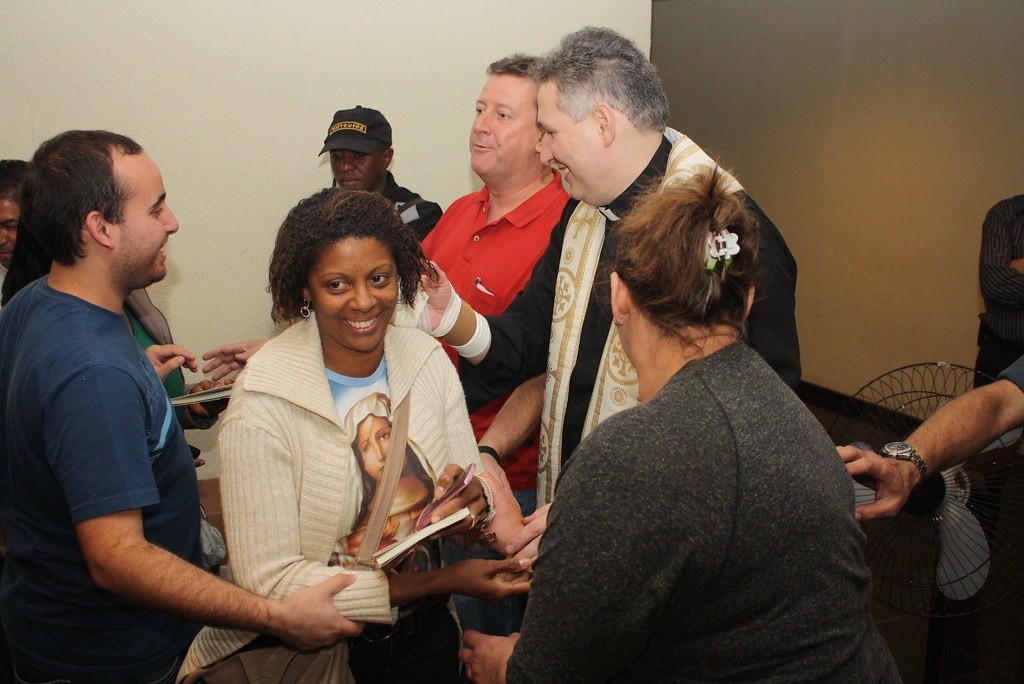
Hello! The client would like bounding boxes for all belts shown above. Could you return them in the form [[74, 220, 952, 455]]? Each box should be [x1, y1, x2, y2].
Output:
[[347, 598, 443, 643]]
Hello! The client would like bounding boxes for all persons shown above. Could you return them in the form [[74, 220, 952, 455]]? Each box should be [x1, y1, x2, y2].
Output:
[[389, 26, 802, 570], [319, 107, 443, 242], [973, 194, 1024, 389], [0, 130, 365, 684], [836, 355, 1024, 521], [218, 188, 532, 684], [458, 168, 902, 684], [420, 57, 571, 684]]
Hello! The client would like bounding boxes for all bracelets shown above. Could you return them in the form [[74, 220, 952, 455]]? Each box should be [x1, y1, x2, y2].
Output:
[[478, 446, 500, 464], [474, 474, 497, 542]]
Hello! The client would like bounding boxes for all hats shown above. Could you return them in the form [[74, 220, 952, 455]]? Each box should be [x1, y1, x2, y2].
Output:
[[318, 105, 393, 156]]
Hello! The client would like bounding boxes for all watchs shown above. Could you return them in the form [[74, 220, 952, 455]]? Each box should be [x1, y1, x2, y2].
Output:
[[880, 442, 928, 481]]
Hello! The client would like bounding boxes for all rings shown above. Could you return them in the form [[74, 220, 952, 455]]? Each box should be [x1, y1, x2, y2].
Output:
[[469, 515, 479, 531], [468, 667, 472, 679]]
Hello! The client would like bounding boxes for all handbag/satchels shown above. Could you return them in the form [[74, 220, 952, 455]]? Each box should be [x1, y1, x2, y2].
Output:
[[182, 635, 356, 684]]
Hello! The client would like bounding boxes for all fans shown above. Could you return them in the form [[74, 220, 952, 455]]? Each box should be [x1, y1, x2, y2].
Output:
[[829, 364, 1024, 684]]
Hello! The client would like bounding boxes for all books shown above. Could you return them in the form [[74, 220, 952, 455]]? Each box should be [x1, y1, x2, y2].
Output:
[[372, 508, 470, 569], [169, 383, 233, 406]]
